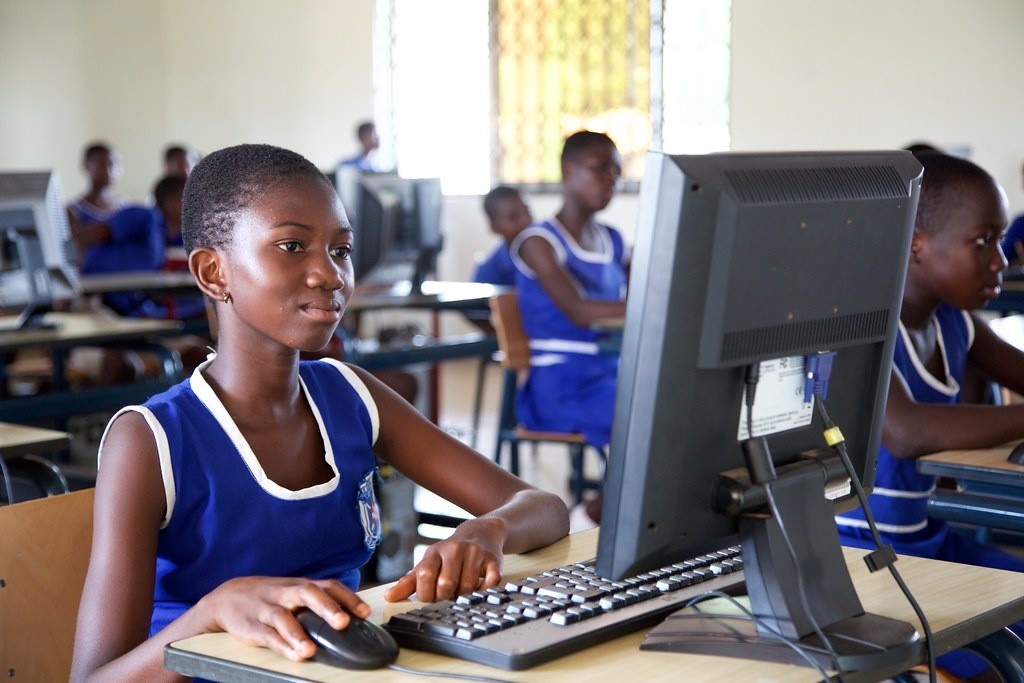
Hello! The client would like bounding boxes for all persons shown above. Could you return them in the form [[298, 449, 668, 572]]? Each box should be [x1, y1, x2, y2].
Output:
[[1001, 160, 1024, 270], [68, 144, 571, 683], [66, 121, 628, 524], [834, 144, 1024, 683]]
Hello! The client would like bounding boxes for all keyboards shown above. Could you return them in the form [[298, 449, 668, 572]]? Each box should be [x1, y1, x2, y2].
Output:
[[380, 545, 746, 672]]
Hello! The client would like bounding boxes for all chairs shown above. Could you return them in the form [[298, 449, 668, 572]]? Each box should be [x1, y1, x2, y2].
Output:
[[0, 486, 95, 683], [489, 292, 610, 504]]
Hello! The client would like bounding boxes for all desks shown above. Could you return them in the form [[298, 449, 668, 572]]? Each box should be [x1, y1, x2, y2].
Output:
[[163, 527, 1024, 683], [342, 278, 509, 453], [81, 272, 197, 319], [0, 312, 185, 463], [0, 421, 72, 459], [917, 438, 1024, 531]]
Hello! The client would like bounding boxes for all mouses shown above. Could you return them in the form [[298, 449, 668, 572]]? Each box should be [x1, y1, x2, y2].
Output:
[[1008, 441, 1024, 466], [296, 610, 400, 671]]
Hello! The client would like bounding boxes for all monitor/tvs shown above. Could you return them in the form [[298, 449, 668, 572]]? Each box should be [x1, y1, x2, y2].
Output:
[[1, 170, 83, 333], [594, 149, 923, 673], [356, 177, 444, 299]]
